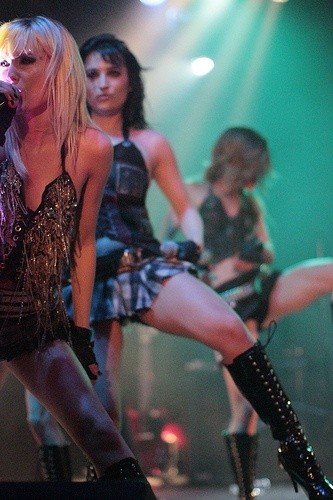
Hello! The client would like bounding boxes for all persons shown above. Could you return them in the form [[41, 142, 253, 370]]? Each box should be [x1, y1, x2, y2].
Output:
[[24, 388, 99, 484], [157, 125, 333, 500], [61, 34, 333, 500], [0, 17, 159, 500]]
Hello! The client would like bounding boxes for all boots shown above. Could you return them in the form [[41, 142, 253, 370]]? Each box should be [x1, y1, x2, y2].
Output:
[[38, 442, 72, 481], [96, 457, 157, 500], [220, 320, 333, 500], [222, 430, 260, 500], [83, 461, 97, 481]]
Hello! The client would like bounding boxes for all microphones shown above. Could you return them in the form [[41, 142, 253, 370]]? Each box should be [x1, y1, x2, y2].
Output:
[[159, 240, 199, 262], [0, 85, 19, 111]]
